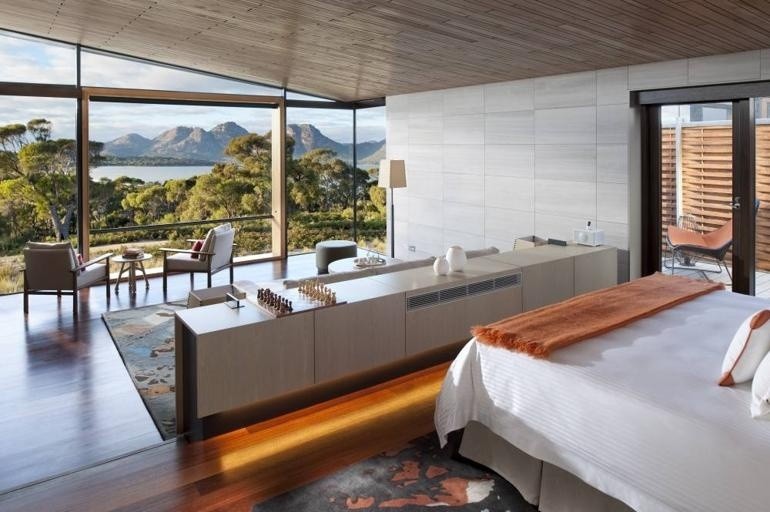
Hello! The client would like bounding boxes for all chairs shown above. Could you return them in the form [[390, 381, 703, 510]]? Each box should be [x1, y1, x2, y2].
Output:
[[664, 198, 760, 285], [157, 223, 235, 302], [18, 241, 113, 315]]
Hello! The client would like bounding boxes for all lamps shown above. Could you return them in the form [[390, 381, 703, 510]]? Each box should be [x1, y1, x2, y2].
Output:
[[377, 158, 408, 258]]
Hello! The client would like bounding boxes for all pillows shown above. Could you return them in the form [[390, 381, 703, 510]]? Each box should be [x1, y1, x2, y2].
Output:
[[190, 240, 204, 257], [76, 254, 85, 272], [714, 309, 769, 421]]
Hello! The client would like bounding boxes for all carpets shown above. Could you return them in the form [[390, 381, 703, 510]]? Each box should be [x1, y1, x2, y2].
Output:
[[100, 299, 189, 442]]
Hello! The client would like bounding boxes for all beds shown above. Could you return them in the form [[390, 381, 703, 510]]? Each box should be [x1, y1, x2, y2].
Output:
[[460, 271, 768, 512]]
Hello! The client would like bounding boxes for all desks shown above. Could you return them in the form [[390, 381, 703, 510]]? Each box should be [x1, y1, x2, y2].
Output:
[[328, 256, 401, 274], [112, 253, 153, 296]]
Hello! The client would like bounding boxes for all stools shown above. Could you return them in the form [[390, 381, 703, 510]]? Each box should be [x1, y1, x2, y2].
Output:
[[314, 239, 358, 275], [187, 285, 247, 310]]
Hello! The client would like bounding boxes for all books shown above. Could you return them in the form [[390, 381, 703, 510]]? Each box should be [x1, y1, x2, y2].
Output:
[[122, 247, 146, 259]]
